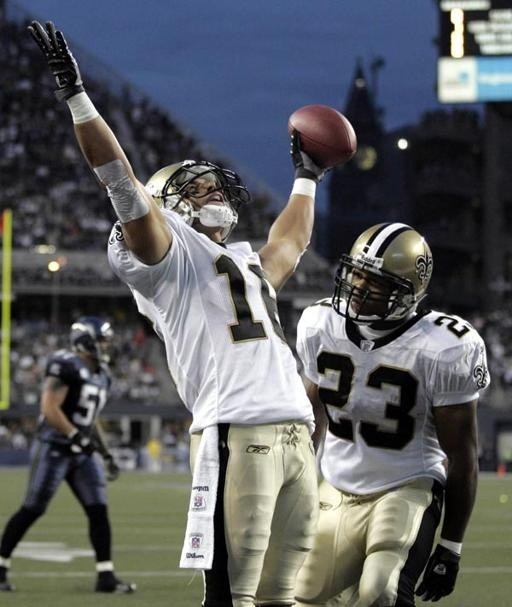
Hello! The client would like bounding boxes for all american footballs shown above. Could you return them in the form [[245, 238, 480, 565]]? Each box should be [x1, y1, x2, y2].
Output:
[[288, 104, 357, 164]]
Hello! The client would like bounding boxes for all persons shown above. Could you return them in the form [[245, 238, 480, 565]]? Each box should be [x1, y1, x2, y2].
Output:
[[1, 13, 512, 462], [1, 320, 141, 596], [20, 17, 325, 606], [296, 221, 492, 606]]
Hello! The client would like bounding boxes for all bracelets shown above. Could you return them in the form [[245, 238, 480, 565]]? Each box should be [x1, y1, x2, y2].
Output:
[[290, 177, 319, 200], [65, 92, 100, 123], [67, 427, 78, 440], [438, 538, 465, 557]]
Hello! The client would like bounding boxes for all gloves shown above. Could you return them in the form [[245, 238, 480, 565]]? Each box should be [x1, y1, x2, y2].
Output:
[[414, 543, 461, 602], [102, 453, 120, 482], [290, 129, 332, 186], [69, 429, 98, 456], [28, 19, 85, 102]]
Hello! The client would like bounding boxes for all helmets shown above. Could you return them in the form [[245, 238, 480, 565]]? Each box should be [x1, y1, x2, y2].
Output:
[[144, 159, 253, 242], [331, 221, 434, 323], [70, 317, 113, 365]]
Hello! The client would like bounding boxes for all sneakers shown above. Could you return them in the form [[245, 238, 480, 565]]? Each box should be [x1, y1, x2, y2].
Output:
[[95, 570, 137, 593], [0, 567, 14, 590]]
